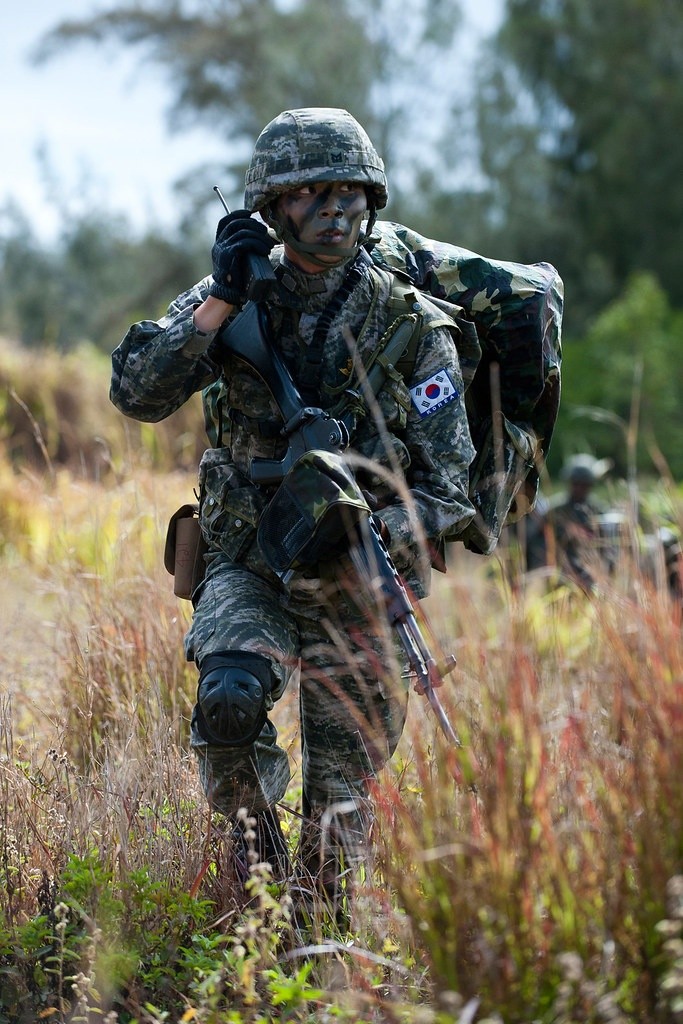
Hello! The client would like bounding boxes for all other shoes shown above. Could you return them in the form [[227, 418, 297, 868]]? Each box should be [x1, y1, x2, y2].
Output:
[[293, 852, 354, 937], [233, 808, 289, 887]]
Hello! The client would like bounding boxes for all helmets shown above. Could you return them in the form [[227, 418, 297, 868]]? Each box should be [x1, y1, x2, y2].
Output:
[[243, 106, 390, 214]]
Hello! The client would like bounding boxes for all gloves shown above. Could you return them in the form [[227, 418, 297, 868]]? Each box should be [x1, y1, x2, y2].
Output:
[[316, 515, 382, 561], [211, 207, 277, 308]]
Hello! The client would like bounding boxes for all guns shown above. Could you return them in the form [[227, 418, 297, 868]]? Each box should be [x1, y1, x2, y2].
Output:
[[204, 296, 478, 802]]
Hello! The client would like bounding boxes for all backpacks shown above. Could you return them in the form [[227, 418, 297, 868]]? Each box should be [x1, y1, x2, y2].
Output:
[[362, 220, 564, 555]]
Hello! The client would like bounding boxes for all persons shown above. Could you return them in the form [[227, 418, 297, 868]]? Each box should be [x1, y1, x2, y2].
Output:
[[527, 454, 618, 594], [110, 107, 476, 959]]
